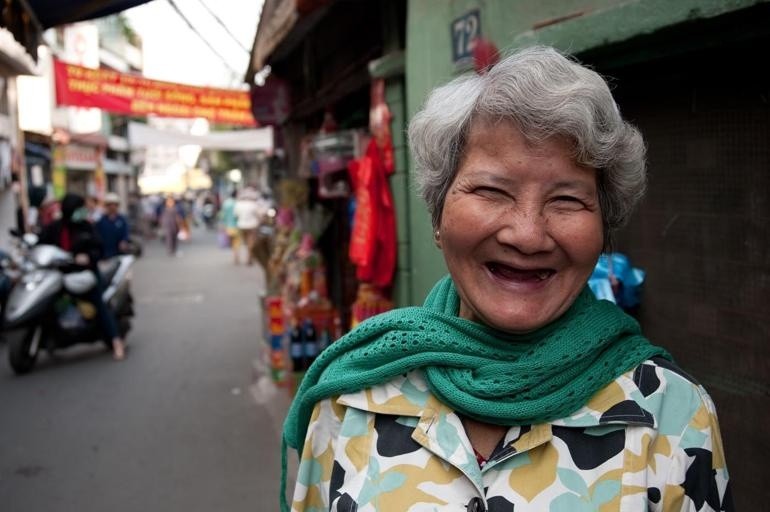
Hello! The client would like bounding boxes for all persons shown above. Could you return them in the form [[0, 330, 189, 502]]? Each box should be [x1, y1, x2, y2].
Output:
[[93, 190, 132, 257], [35, 190, 127, 364], [277, 42, 738, 512], [137, 188, 219, 256]]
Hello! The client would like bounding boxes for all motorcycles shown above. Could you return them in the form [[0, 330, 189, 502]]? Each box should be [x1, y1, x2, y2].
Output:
[[3, 226, 132, 375]]
